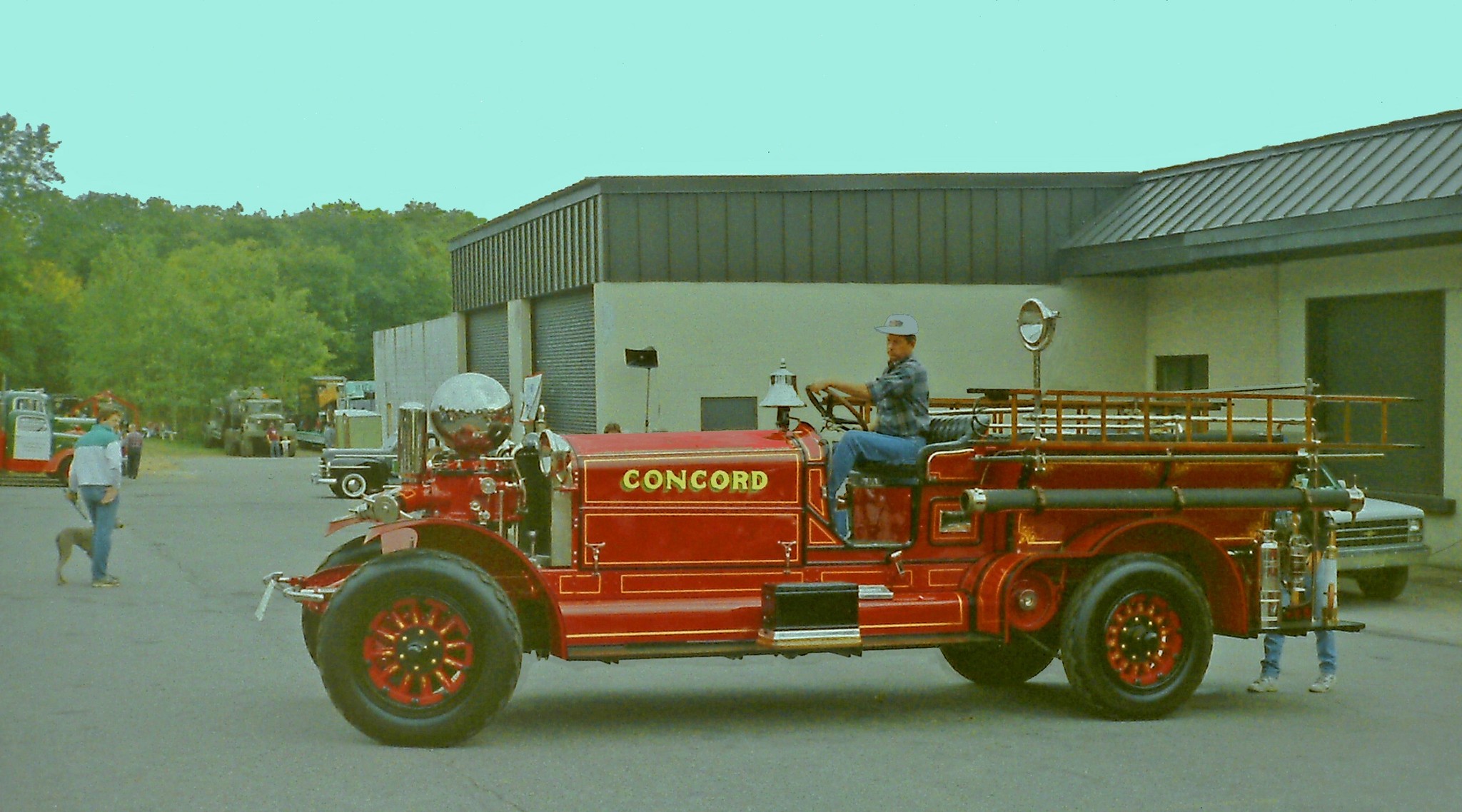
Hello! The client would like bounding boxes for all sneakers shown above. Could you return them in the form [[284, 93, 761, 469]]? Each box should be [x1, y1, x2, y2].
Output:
[[1309, 675, 1338, 691], [92, 575, 120, 587], [1248, 676, 1279, 692]]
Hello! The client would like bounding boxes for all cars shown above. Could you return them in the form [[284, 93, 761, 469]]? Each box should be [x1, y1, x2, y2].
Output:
[[1292, 449, 1430, 601], [311, 428, 518, 499]]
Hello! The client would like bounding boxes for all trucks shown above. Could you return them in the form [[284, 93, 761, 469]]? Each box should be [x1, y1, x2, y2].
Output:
[[204, 390, 299, 458], [256, 353, 1428, 750], [0, 386, 142, 490]]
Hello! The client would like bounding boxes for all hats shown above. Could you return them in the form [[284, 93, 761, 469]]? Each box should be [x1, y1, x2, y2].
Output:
[[875, 314, 918, 335]]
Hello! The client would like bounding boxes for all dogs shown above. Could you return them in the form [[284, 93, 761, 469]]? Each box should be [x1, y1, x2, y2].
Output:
[[54, 518, 123, 587]]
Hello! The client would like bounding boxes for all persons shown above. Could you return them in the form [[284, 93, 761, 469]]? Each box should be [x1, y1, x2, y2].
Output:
[[1246, 505, 1339, 695], [69, 408, 122, 586], [122, 424, 143, 478], [266, 422, 281, 458], [811, 316, 931, 512]]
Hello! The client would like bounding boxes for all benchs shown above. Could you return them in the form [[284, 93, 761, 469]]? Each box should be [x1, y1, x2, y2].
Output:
[[871, 410, 984, 482]]
